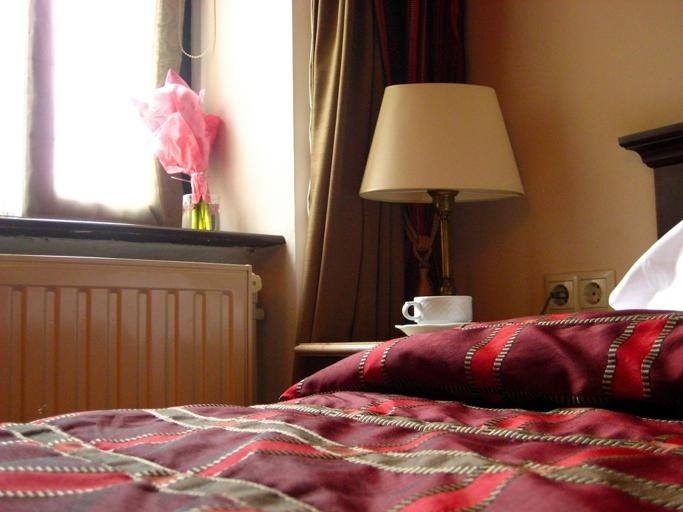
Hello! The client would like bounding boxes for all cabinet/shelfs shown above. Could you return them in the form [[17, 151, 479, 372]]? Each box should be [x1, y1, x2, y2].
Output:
[[180, 193, 193, 230], [210, 203, 219, 231]]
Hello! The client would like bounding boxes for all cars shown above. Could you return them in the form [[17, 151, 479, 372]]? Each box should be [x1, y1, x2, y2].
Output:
[[400, 294, 473, 323]]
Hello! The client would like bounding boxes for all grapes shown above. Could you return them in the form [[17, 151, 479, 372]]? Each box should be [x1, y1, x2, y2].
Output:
[[288, 341, 379, 380]]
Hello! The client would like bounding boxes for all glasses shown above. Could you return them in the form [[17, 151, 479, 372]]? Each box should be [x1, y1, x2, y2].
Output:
[[358, 81, 528, 295]]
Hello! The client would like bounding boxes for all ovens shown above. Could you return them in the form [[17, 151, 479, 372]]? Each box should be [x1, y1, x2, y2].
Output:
[[1, 112, 683, 511]]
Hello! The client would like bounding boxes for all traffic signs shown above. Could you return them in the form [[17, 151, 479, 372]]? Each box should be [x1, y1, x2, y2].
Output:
[[580, 270, 616, 310], [542, 271, 579, 311]]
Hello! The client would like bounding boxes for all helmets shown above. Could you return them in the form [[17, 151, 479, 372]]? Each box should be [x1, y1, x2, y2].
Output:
[[391, 323, 466, 336]]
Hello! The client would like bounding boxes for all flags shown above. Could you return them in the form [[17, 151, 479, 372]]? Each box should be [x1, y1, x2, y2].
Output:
[[190, 173, 217, 230]]
